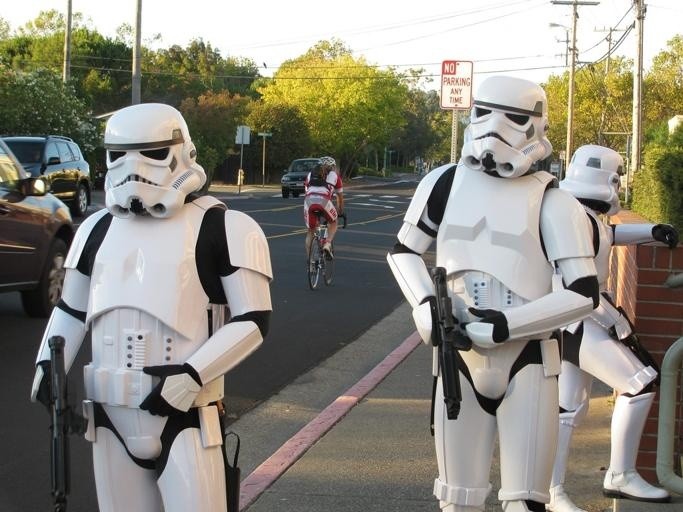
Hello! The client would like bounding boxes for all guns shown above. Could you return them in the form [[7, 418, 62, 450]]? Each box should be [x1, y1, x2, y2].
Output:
[[601, 291, 660, 387], [431, 267, 472, 419], [47, 336, 88, 512]]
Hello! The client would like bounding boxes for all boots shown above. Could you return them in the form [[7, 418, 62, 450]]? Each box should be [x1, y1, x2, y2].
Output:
[[602, 392, 672, 503], [547, 412, 589, 512]]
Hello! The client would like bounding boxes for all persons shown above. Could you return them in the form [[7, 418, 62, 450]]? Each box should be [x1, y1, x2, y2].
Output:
[[387, 76, 599, 512], [31, 102, 274, 512], [304, 156, 344, 273], [545, 144, 679, 512]]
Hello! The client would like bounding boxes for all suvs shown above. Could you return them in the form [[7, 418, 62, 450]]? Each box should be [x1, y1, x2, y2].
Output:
[[0, 137, 75, 319], [280, 157, 318, 199], [2, 135, 92, 218]]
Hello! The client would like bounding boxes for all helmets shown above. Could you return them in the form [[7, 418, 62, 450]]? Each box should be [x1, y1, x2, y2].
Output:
[[559, 142, 626, 217], [320, 155, 336, 167], [101, 101, 207, 221], [458, 77, 554, 182]]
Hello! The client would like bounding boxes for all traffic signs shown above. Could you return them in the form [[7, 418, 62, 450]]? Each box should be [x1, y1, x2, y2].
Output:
[[439, 60, 474, 110]]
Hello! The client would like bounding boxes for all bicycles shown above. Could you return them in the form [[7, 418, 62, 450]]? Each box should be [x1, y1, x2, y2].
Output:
[[305, 203, 347, 291]]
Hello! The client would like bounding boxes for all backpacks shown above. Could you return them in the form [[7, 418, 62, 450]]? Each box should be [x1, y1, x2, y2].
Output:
[[307, 166, 336, 188]]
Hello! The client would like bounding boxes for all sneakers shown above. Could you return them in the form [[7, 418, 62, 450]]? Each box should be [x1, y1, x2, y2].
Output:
[[322, 243, 334, 261]]
